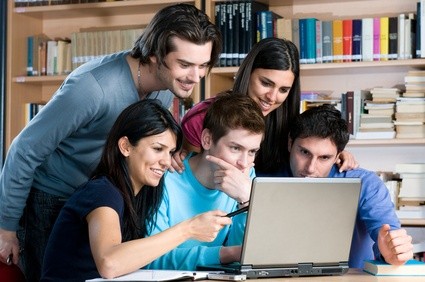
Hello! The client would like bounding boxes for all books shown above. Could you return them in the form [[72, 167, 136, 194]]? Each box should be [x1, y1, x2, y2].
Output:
[[362, 259, 425, 274], [25, 26, 146, 76], [23, 100, 46, 127], [85, 269, 224, 280], [299, 70, 424, 141], [213, 0, 425, 67], [376, 163, 424, 225]]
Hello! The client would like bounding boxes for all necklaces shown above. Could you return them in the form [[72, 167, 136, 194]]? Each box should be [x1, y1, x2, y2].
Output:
[[136, 60, 159, 103]]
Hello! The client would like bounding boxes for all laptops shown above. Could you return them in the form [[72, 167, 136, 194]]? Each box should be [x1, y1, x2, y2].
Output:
[[196, 176, 364, 277]]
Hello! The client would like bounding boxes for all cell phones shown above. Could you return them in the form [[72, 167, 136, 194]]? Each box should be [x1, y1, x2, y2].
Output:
[[206, 272, 247, 281]]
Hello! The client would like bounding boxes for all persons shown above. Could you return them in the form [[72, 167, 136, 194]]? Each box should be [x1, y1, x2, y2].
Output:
[[0, 1, 222, 280], [40, 98, 233, 282], [167, 36, 360, 175], [270, 101, 414, 269], [143, 88, 266, 272]]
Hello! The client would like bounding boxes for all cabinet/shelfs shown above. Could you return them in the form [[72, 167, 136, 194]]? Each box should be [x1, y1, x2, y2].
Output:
[[203, 0, 424, 260], [5, 1, 201, 177]]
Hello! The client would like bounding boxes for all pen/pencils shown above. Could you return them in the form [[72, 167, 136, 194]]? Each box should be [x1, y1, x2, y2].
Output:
[[227, 206, 249, 218]]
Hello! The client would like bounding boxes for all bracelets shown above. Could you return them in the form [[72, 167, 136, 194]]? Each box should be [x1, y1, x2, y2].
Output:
[[236, 200, 249, 205]]
